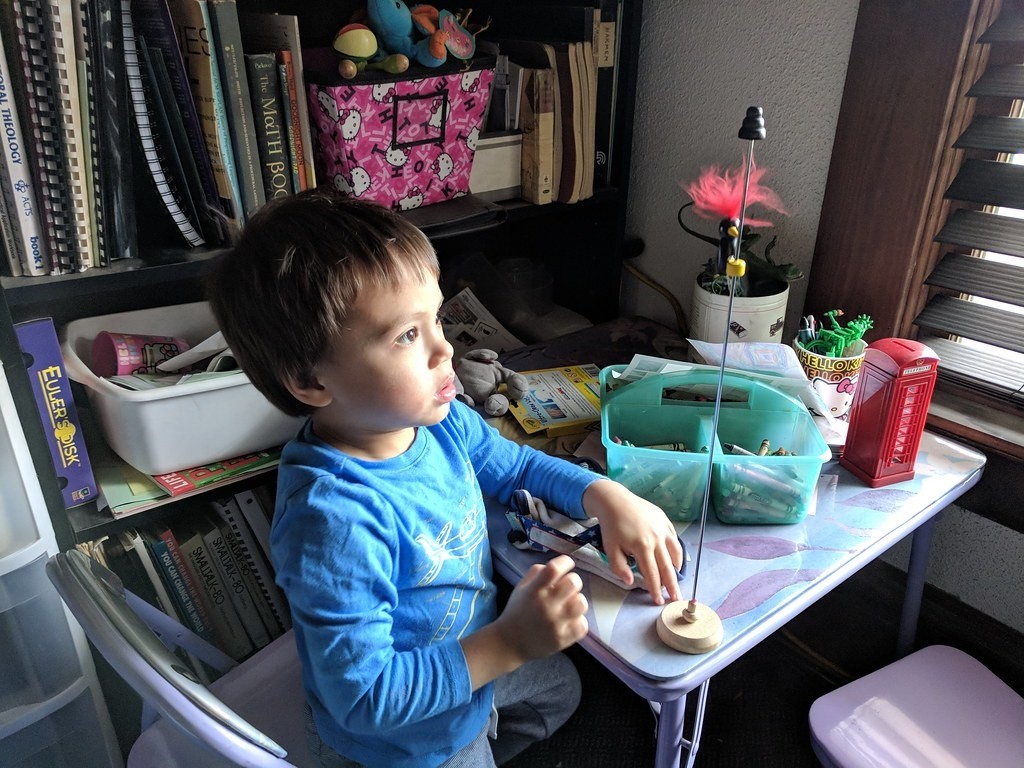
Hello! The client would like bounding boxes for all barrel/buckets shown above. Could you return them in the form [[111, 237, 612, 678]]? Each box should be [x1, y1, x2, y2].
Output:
[[689, 272, 791, 360]]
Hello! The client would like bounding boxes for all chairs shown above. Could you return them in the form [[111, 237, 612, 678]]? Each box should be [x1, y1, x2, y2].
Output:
[[45, 548, 360, 768]]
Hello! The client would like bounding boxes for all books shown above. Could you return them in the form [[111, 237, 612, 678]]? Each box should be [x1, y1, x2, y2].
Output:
[[74, 468, 291, 687], [0, 0, 317, 279], [483, 2, 614, 203]]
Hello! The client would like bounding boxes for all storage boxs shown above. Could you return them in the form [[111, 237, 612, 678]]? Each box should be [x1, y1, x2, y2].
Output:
[[304, 63, 498, 216], [12, 303, 100, 510], [59, 299, 310, 476], [468, 133, 522, 194]]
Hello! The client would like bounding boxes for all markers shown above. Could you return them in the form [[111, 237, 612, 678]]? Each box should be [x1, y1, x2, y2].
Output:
[[798, 315, 823, 353]]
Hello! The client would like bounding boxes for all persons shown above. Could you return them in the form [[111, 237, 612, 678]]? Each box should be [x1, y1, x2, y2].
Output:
[[222, 188, 685, 768]]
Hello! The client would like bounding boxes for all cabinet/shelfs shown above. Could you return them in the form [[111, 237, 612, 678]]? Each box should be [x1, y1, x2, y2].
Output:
[[0, 358, 125, 768], [0, 1, 642, 768]]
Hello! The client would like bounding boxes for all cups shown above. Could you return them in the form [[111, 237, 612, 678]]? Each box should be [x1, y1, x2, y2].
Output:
[[792, 331, 867, 415], [92, 329, 192, 378]]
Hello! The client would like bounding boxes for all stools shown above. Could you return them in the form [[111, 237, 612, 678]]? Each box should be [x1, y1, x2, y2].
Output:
[[808, 645, 1024, 767]]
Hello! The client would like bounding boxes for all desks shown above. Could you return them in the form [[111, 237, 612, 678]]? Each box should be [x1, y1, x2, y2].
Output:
[[470, 316, 987, 768]]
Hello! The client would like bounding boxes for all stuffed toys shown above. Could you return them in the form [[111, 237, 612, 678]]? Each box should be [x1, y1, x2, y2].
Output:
[[364, 0, 450, 68], [450, 348, 529, 414]]
